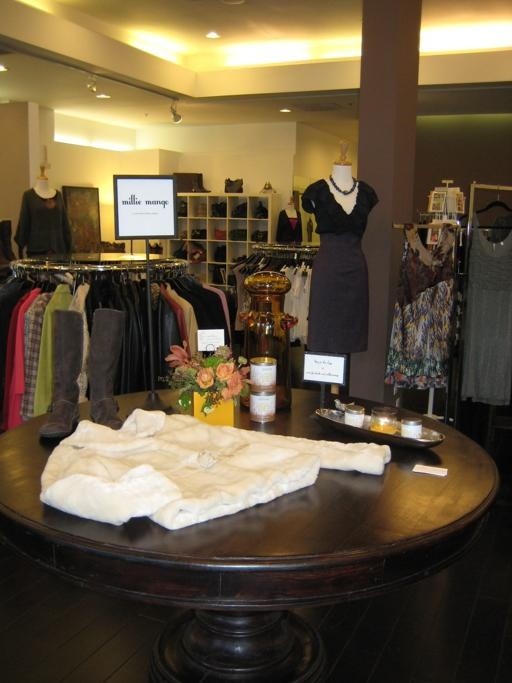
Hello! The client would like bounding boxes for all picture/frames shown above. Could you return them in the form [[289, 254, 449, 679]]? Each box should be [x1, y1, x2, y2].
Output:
[[62, 186, 101, 253], [113, 174, 179, 239], [301, 351, 347, 386]]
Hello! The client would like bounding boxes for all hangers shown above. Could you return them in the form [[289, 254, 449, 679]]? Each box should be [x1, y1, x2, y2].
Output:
[[459, 184, 512, 230], [230, 244, 318, 277], [9, 258, 194, 296]]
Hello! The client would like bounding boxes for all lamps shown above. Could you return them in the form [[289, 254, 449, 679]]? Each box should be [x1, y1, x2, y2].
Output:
[[169, 98, 182, 123]]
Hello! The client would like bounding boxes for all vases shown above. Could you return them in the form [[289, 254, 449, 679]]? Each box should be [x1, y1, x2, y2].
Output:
[[193, 392, 241, 429]]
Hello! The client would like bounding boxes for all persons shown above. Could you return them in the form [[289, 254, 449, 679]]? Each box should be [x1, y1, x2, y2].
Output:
[[14, 174, 73, 258], [301, 159, 380, 354]]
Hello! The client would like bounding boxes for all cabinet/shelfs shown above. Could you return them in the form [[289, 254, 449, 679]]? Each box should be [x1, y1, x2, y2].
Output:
[[166, 193, 278, 290]]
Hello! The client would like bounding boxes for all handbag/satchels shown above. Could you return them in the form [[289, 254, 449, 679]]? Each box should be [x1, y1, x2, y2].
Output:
[[232, 203, 247, 218], [225, 178, 243, 194], [212, 202, 226, 217], [198, 204, 206, 217], [230, 229, 246, 241], [178, 201, 187, 216], [190, 247, 206, 264], [214, 246, 226, 262], [179, 230, 187, 239], [191, 229, 206, 239], [215, 229, 226, 240], [228, 274, 236, 286], [251, 229, 267, 242], [174, 248, 187, 259], [254, 202, 268, 219], [213, 267, 226, 282]]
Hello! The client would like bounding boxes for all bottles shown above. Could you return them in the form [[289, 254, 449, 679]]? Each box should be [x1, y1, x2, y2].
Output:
[[344, 404, 422, 439], [241, 273, 293, 423]]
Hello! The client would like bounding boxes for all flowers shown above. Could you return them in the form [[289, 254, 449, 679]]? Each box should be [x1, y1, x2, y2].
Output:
[[159, 340, 250, 414]]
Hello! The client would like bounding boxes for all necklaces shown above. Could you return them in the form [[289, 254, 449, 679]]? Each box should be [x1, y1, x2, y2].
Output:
[[329, 173, 357, 195]]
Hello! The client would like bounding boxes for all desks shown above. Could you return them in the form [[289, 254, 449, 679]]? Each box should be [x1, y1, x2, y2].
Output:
[[0, 388, 500, 683]]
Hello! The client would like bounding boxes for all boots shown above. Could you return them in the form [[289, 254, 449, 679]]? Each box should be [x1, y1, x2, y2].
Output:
[[192, 175, 206, 192], [88, 309, 125, 429], [39, 311, 83, 437]]
